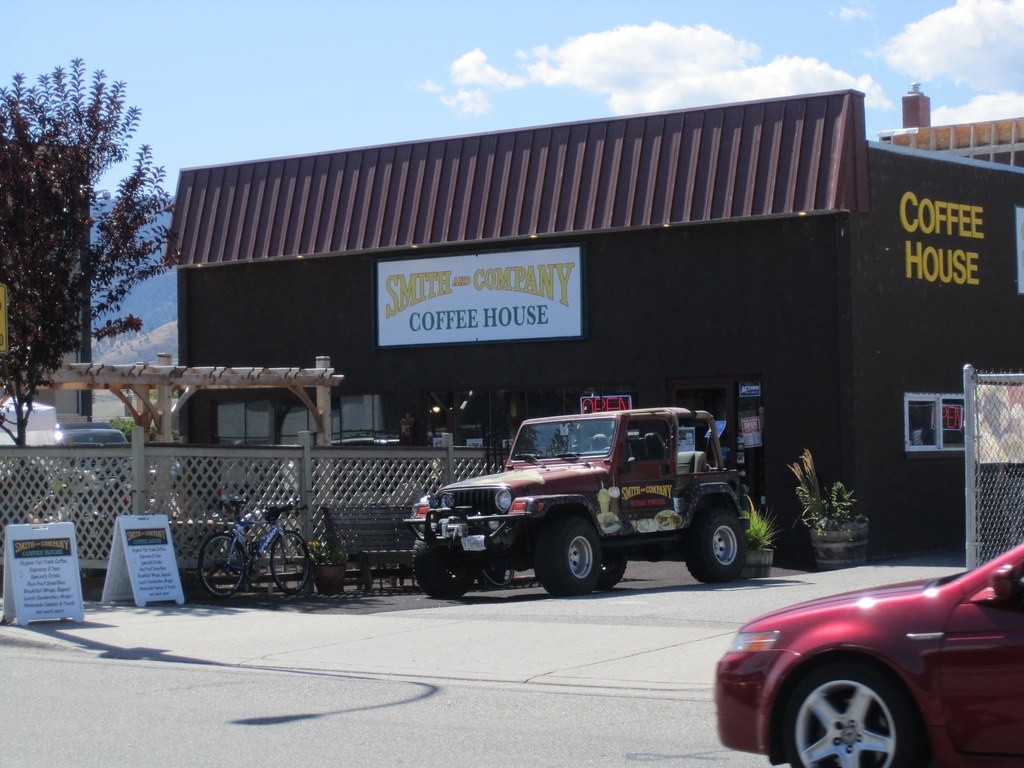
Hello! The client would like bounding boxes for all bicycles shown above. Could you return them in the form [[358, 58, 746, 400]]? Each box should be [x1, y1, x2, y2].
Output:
[[198, 497, 311, 598]]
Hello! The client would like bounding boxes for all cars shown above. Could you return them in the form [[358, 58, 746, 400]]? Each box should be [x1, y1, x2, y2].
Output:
[[60, 428, 133, 481], [712, 541, 1024, 768]]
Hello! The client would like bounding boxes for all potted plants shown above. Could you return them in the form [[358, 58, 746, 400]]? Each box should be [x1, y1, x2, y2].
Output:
[[739, 494, 782, 580], [786, 447, 869, 570]]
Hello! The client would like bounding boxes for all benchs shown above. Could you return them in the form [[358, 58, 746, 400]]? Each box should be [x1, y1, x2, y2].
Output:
[[322, 502, 419, 593]]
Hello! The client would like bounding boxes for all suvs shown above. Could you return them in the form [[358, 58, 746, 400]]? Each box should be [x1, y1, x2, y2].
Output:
[[402, 406, 751, 600]]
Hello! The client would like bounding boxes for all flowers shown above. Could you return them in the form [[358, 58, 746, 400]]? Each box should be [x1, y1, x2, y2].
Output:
[[307, 539, 350, 566]]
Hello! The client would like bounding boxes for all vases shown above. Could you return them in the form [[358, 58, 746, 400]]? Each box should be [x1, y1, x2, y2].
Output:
[[312, 563, 346, 596]]
